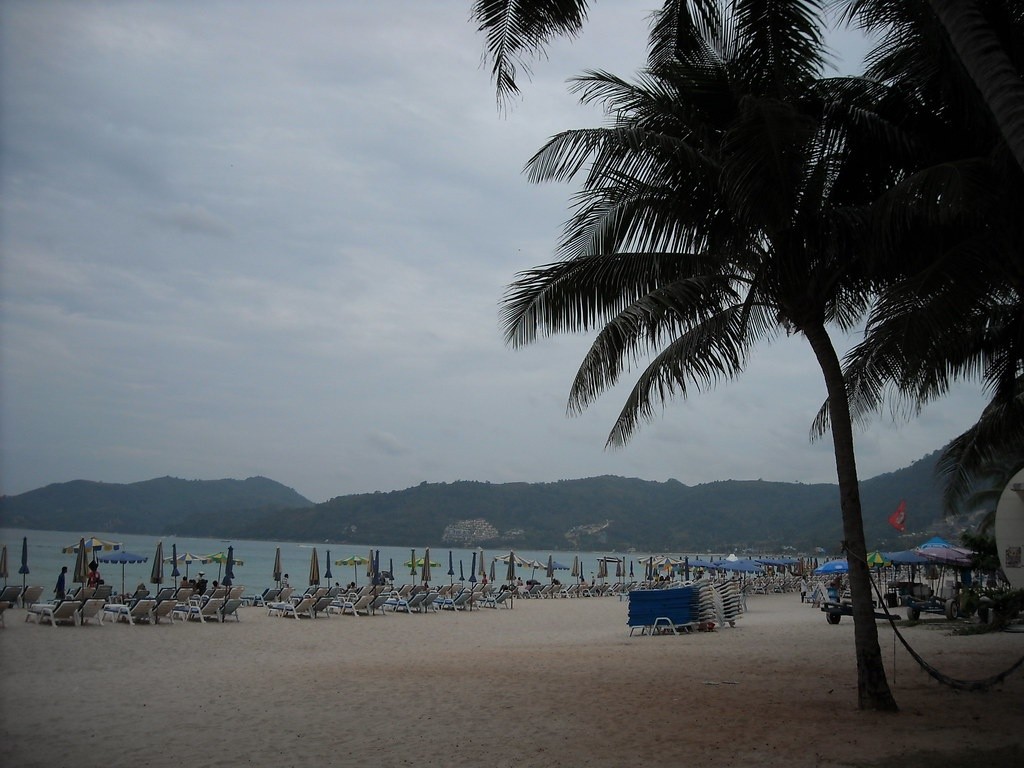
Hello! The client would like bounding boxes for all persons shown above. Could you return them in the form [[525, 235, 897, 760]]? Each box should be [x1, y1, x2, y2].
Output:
[[281, 573, 288, 588], [660, 575, 664, 581], [592, 576, 595, 585], [482, 575, 487, 584], [801, 575, 808, 603], [126, 583, 146, 597], [86, 560, 101, 588], [351, 582, 356, 589], [340, 584, 351, 594], [499, 584, 516, 592], [54, 566, 68, 599], [665, 575, 670, 581], [517, 577, 521, 586], [181, 571, 220, 595], [695, 571, 701, 581]]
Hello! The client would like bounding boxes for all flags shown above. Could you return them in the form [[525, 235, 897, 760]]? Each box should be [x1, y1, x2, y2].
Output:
[[888, 500, 906, 531]]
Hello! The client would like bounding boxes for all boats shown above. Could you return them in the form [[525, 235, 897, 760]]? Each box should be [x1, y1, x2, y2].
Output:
[[596, 556, 622, 562]]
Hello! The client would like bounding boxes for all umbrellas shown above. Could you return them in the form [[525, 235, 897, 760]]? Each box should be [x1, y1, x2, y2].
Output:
[[274, 546, 634, 616], [221, 546, 235, 623], [171, 543, 180, 590], [814, 558, 849, 579], [866, 535, 980, 606], [164, 552, 206, 579], [0, 535, 165, 626], [638, 555, 841, 596], [203, 552, 243, 585]]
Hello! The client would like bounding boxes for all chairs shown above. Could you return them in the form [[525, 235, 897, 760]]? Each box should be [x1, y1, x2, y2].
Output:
[[0, 568, 924, 636]]
